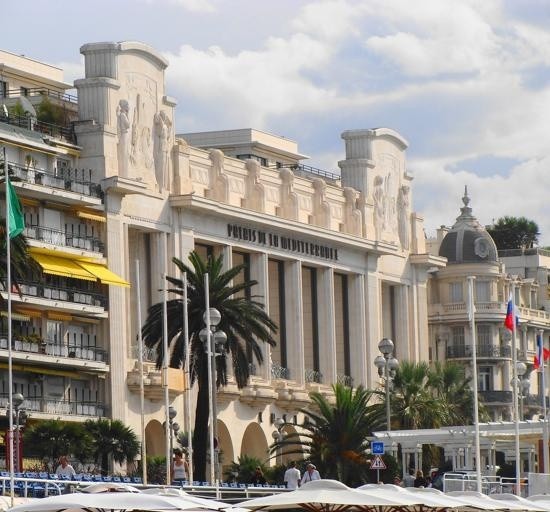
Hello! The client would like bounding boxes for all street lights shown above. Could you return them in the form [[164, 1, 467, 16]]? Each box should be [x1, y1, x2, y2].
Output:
[[373, 336, 400, 454], [5, 392, 30, 472], [198, 305, 229, 483], [163, 406, 180, 484], [509, 360, 530, 421], [270, 416, 288, 469]]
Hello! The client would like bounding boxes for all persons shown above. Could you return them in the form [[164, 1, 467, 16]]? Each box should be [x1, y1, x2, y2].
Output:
[[116, 98, 139, 181], [300, 463, 321, 486], [55, 456, 75, 476], [430, 471, 443, 491], [153, 109, 173, 193], [402, 468, 416, 487], [423, 475, 433, 488], [393, 476, 402, 487], [285, 460, 301, 492], [173, 451, 191, 482], [414, 470, 425, 487]]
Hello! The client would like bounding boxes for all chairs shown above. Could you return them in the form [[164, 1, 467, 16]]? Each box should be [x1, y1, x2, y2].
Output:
[[0, 472, 288, 500]]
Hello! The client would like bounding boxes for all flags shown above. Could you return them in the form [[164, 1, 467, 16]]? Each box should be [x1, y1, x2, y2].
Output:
[[534, 332, 550, 368], [7, 166, 25, 240], [503, 289, 521, 333], [464, 283, 477, 323]]
